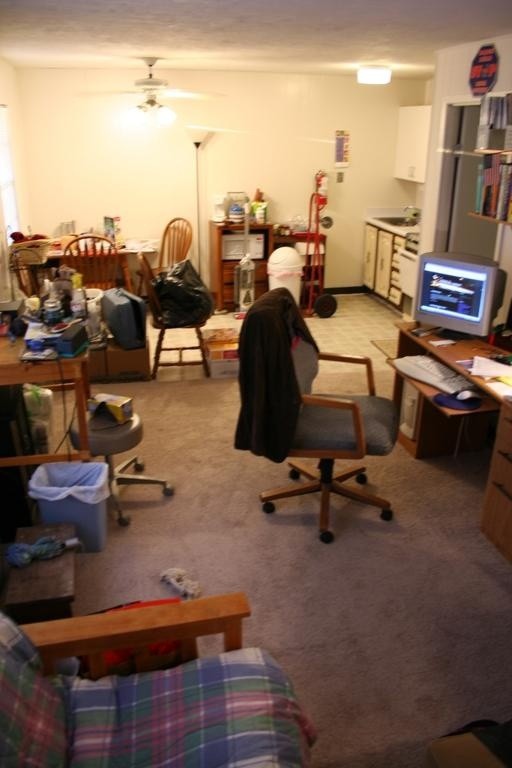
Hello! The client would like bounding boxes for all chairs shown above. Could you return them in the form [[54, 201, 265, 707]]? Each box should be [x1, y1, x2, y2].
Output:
[[61, 216, 210, 380], [242, 288, 400, 545], [0, 590, 309, 768]]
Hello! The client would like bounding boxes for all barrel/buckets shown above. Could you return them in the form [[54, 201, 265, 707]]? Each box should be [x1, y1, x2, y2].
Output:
[[83, 287, 104, 338]]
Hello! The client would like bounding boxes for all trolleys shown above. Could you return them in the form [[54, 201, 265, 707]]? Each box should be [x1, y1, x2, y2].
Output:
[[298, 168, 337, 318]]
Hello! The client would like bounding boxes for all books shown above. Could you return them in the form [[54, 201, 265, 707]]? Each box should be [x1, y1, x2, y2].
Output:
[[487, 91, 512, 153], [474, 156, 512, 224]]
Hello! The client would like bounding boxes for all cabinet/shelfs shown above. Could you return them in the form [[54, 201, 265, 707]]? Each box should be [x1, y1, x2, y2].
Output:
[[361, 223, 407, 306], [467, 146, 511, 227], [207, 220, 327, 311]]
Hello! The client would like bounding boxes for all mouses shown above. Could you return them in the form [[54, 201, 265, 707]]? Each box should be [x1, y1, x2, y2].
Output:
[[456, 390, 481, 402]]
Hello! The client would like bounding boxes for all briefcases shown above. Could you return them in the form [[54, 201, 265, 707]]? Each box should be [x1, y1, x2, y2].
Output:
[[102, 287, 147, 351]]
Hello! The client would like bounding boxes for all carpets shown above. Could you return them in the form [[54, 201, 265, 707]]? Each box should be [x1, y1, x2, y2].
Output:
[[369, 337, 397, 359]]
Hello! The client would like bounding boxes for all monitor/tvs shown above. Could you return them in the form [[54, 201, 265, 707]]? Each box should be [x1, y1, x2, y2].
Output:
[[413, 252, 507, 340]]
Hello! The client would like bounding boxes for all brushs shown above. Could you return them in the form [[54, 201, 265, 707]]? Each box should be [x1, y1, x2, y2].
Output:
[[51, 318, 83, 333]]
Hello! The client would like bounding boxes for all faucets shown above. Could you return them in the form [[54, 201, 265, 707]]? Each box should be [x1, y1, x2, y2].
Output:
[[403, 205, 421, 212]]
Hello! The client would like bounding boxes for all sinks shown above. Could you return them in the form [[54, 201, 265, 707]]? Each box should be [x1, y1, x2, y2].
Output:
[[373, 216, 419, 227]]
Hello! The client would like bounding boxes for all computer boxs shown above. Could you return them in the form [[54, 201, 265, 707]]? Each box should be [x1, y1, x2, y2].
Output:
[[398, 379, 419, 440]]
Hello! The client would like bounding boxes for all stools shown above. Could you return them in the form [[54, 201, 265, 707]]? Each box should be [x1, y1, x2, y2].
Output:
[[68, 411, 174, 527]]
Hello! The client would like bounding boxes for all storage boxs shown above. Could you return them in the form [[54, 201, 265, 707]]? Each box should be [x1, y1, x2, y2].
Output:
[[81, 339, 154, 389]]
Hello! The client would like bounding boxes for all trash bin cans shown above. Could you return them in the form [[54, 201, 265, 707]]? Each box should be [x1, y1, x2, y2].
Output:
[[28, 462, 109, 553], [267, 246, 302, 307]]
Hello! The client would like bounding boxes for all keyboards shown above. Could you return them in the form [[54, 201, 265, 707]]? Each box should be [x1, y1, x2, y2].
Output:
[[392, 355, 475, 396]]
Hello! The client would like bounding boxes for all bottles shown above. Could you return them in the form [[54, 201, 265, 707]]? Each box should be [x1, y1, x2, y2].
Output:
[[43, 289, 87, 327], [230, 205, 244, 225]]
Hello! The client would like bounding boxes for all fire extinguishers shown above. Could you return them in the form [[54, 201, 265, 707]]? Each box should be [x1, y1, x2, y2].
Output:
[[316, 169, 329, 205]]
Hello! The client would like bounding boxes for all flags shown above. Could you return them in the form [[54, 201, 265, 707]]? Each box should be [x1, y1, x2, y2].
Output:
[[483, 153, 500, 186]]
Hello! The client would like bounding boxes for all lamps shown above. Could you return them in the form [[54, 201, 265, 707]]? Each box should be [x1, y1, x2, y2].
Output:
[[185, 128, 207, 279]]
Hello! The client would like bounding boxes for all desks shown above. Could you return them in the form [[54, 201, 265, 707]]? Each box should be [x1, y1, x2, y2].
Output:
[[0, 336, 90, 468], [386, 322, 511, 566]]
